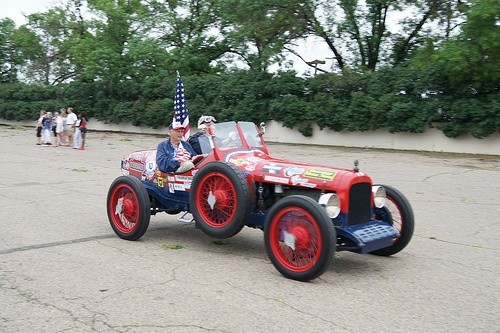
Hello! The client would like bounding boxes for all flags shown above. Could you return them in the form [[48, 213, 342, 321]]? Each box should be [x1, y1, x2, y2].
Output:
[[173, 74, 191, 142]]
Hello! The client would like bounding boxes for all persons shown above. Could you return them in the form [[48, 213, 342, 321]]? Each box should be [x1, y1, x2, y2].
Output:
[[188, 116, 235, 157], [36, 107, 87, 150], [156, 122, 198, 174]]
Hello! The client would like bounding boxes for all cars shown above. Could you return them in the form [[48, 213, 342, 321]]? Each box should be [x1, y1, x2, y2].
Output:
[[106, 119, 415, 282]]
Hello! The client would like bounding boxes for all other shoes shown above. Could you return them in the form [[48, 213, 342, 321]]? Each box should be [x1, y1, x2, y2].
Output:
[[46, 143, 51, 145], [37, 143, 41, 145], [79, 147, 84, 150], [74, 146, 78, 149]]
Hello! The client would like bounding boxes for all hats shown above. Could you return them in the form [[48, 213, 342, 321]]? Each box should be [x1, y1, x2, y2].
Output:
[[168, 122, 185, 130], [198, 116, 215, 130]]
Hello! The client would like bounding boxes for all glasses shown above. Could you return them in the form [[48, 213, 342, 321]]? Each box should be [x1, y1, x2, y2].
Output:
[[200, 117, 217, 125]]
